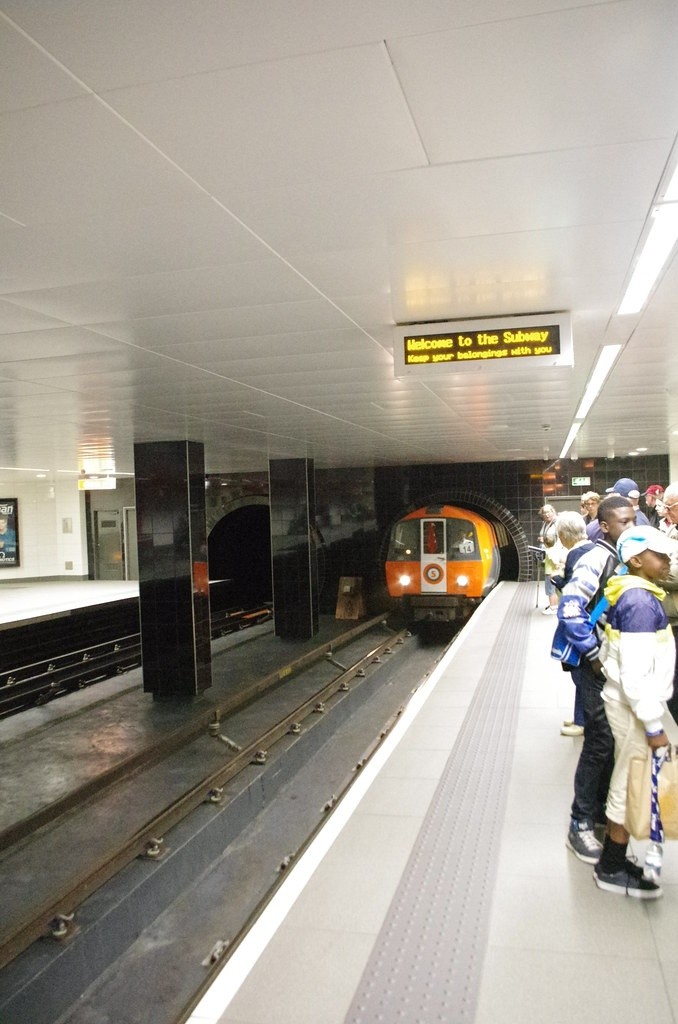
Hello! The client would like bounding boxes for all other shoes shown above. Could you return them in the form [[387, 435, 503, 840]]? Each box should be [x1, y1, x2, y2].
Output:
[[562, 725, 585, 736], [564, 720, 576, 725], [542, 607, 558, 615]]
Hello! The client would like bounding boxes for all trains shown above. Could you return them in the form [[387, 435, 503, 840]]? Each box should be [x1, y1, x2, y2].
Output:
[[385, 503, 508, 621]]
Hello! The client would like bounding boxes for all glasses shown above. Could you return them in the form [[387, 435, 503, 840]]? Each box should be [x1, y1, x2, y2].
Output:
[[664, 502, 678, 511]]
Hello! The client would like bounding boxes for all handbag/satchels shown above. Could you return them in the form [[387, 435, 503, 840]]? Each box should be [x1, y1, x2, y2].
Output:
[[625, 743, 678, 841]]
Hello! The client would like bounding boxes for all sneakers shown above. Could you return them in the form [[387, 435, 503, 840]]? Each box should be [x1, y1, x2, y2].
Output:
[[565, 820, 604, 864], [594, 861, 655, 884], [597, 867, 663, 898]]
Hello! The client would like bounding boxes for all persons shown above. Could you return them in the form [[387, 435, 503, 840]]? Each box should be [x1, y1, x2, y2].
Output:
[[593, 525, 678, 899], [537, 505, 569, 615], [641, 482, 678, 540], [0, 516, 16, 549], [580, 491, 600, 525], [550, 496, 635, 863], [555, 511, 596, 735], [605, 478, 650, 526], [450, 529, 475, 553]]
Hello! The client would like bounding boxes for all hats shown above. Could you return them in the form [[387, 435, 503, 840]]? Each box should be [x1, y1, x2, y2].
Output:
[[617, 525, 678, 564], [641, 484, 664, 496], [606, 478, 640, 499]]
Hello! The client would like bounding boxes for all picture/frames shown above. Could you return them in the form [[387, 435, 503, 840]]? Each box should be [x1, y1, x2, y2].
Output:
[[0, 497, 22, 566]]
